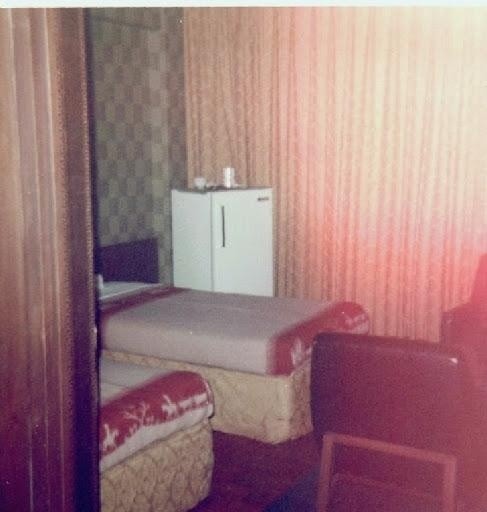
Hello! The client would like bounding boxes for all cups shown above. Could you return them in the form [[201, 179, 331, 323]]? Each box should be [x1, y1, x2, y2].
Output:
[[223, 168, 235, 188], [193, 177, 206, 189]]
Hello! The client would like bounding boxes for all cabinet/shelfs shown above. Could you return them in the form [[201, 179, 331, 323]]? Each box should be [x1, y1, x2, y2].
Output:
[[170, 184, 275, 299]]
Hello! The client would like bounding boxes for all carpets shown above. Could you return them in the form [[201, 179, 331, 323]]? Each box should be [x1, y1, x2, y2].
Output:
[[265, 466, 316, 510]]
